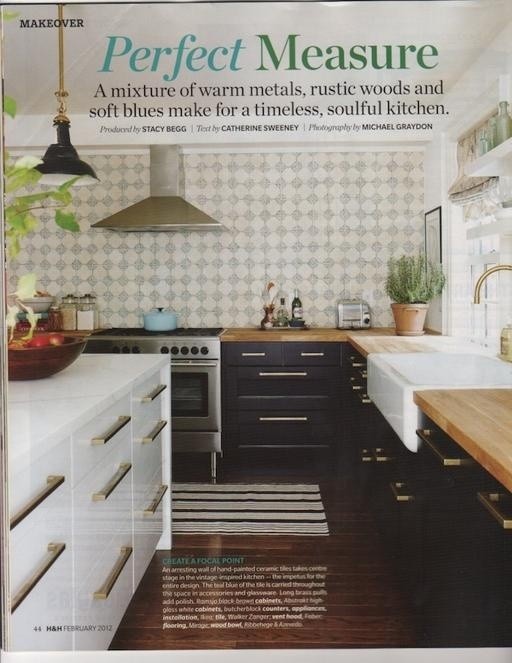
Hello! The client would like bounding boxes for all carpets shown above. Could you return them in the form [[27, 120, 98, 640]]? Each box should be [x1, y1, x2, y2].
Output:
[[171, 482, 330, 537]]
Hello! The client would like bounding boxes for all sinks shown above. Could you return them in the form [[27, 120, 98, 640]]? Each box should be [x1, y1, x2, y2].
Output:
[[367, 351, 512, 453]]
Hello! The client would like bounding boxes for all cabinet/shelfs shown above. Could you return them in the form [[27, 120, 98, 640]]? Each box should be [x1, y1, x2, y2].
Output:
[[7, 420, 73, 651], [73, 379, 134, 650], [222, 339, 344, 473], [133, 356, 172, 591]]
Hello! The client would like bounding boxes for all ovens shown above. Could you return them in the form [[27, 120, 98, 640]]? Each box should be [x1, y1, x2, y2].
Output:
[[170, 362, 220, 451]]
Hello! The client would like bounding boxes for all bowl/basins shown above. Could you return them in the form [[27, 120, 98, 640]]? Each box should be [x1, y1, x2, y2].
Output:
[[17, 297, 54, 313], [289, 321, 306, 327], [9, 329, 89, 381]]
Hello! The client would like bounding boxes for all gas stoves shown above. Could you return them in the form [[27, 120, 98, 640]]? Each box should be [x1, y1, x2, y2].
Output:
[[83, 326, 222, 359]]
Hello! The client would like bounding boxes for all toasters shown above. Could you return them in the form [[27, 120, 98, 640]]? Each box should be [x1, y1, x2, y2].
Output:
[[337, 298, 371, 330]]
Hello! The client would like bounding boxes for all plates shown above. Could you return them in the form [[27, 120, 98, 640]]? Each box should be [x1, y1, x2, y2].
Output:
[[273, 326, 307, 331]]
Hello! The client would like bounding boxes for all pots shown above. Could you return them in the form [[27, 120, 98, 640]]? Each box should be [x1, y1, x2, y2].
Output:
[[141, 304, 178, 331]]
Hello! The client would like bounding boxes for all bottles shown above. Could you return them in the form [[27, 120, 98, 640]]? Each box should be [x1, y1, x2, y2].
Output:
[[278, 298, 288, 328], [292, 288, 305, 319], [499, 314, 512, 362], [261, 306, 276, 329], [48, 306, 61, 331], [60, 294, 98, 331]]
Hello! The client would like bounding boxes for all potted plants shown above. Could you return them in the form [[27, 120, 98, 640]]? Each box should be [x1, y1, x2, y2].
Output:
[[385, 255, 446, 336]]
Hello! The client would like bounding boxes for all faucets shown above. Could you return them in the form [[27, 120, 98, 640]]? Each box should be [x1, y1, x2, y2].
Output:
[[472, 265, 512, 304]]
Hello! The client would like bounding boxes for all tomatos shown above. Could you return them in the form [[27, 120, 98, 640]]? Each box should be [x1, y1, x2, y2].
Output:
[[29, 334, 65, 345]]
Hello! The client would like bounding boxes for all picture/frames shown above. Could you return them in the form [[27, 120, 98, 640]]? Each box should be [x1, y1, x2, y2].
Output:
[[425, 206, 442, 335]]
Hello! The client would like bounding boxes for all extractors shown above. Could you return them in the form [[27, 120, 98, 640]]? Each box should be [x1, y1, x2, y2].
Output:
[[90, 147, 233, 235]]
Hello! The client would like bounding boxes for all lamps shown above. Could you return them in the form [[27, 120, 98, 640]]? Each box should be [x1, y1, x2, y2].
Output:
[[30, 3, 100, 186]]
